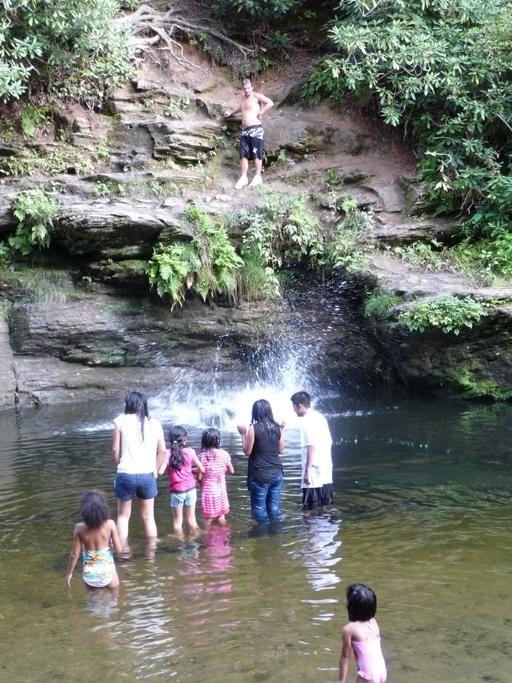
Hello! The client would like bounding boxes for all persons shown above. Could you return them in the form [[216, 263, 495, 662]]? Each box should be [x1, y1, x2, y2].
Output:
[[290, 391, 334, 511], [66, 488, 122, 603], [236, 399, 286, 538], [337, 583, 388, 683], [112, 391, 166, 560], [157, 426, 206, 539], [224, 78, 275, 191], [194, 428, 235, 531]]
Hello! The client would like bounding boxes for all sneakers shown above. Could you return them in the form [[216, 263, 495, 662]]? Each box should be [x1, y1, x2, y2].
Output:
[[248, 175, 262, 187], [234, 175, 249, 189]]
